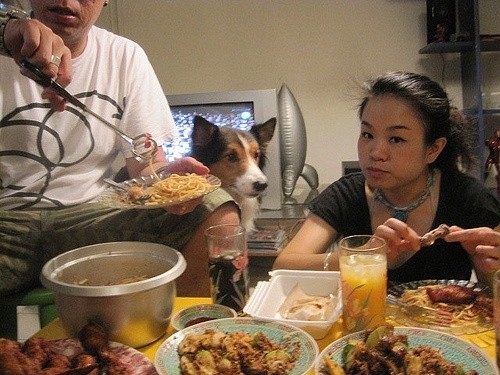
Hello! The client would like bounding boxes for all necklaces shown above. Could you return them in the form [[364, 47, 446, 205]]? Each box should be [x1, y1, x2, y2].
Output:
[[375, 166, 436, 222]]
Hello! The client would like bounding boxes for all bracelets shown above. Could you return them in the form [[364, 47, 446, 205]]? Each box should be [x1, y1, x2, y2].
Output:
[[324, 252, 331, 270]]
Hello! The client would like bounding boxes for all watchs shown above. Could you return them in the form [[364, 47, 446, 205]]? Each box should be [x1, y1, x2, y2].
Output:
[[0, 6, 31, 58]]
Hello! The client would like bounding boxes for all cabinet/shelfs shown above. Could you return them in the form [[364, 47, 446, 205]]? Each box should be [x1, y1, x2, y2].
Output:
[[418, 34, 500, 189]]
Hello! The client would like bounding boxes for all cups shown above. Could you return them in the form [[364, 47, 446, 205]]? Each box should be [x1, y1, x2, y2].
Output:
[[205, 223, 249, 316], [338, 234, 388, 335], [491, 270, 500, 369]]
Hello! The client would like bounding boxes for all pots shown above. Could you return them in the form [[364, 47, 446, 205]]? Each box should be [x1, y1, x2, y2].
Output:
[[38, 241, 186, 349]]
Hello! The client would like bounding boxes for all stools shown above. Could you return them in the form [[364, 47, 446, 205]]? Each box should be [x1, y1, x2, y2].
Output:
[[0, 284, 60, 343]]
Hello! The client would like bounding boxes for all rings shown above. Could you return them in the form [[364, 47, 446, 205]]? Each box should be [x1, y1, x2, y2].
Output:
[[50, 54, 61, 66]]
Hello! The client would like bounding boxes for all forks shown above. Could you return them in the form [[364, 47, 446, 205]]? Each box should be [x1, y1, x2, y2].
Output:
[[385, 295, 452, 327], [103, 177, 153, 201]]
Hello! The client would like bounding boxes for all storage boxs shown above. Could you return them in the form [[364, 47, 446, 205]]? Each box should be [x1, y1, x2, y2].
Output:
[[241, 267, 343, 336]]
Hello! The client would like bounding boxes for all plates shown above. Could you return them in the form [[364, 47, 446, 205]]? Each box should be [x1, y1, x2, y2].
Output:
[[386, 279, 495, 335], [98, 172, 222, 209], [153, 316, 320, 375], [315, 327, 500, 375], [42, 337, 158, 375]]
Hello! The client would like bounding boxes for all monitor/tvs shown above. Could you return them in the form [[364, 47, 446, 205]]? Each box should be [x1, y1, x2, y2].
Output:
[[162, 87, 283, 210]]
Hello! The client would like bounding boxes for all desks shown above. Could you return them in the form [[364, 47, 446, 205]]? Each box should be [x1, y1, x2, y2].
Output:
[[29, 295, 499, 375]]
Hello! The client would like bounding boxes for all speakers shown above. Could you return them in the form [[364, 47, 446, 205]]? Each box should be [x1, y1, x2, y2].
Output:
[[426, 0, 475, 43]]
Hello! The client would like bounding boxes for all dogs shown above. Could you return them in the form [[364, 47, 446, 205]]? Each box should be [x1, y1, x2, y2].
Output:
[[185, 115, 277, 238]]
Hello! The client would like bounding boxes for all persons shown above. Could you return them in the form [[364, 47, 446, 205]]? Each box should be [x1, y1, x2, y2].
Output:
[[272, 72, 500, 297], [0, 0, 243, 335]]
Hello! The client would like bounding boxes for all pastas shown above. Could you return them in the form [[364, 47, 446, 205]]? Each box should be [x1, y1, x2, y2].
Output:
[[142, 155, 211, 202]]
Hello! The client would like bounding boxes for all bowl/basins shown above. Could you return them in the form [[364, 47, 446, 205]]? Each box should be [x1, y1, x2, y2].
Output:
[[171, 304, 238, 333], [243, 269, 343, 340]]
